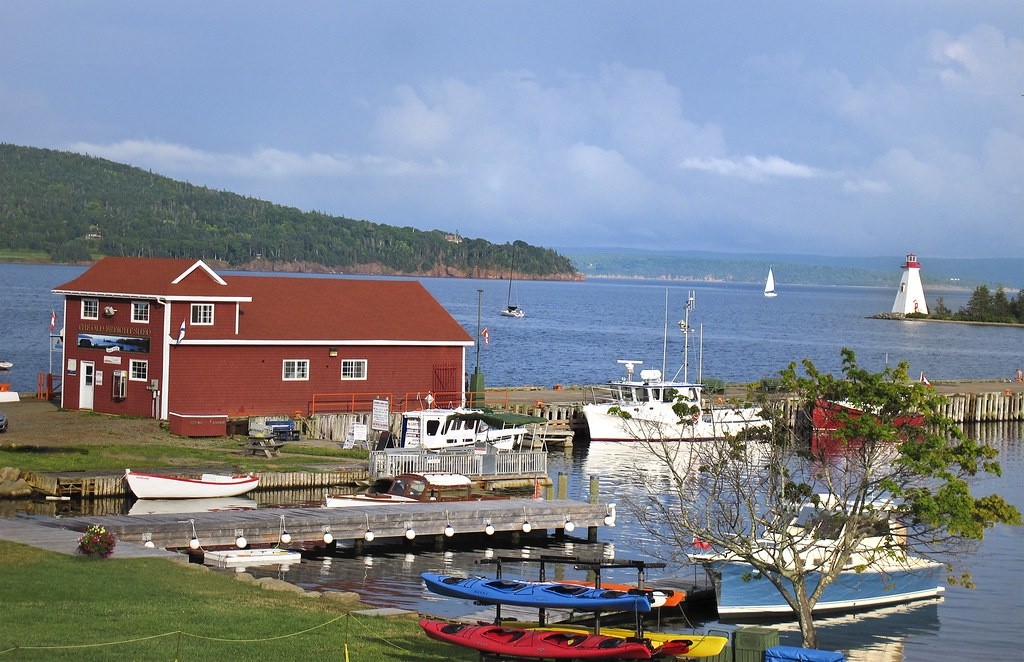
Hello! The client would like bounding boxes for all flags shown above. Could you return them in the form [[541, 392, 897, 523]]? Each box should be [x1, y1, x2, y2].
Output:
[[50, 310, 56, 330], [481, 325, 490, 345], [693, 532, 713, 549], [178, 320, 185, 343], [919, 372, 931, 387]]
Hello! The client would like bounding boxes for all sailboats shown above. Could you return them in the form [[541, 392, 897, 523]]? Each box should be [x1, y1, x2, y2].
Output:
[[501, 250, 524, 318], [763, 269, 778, 297]]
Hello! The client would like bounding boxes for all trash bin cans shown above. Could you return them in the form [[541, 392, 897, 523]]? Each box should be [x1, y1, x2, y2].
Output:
[[734, 627, 779, 662], [700, 624, 742, 662]]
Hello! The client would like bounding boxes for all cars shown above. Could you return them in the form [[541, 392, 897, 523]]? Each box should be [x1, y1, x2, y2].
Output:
[[0, 412, 8, 431]]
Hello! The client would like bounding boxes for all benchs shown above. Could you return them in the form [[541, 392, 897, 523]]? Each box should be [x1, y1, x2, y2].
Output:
[[266, 443, 284, 458], [242, 444, 273, 459], [238, 441, 259, 457]]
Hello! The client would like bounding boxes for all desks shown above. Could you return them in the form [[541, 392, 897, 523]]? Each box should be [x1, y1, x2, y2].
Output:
[[241, 436, 281, 458]]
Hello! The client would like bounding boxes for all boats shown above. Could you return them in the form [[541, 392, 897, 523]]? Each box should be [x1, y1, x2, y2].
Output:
[[491, 618, 730, 659], [586, 286, 778, 441], [394, 402, 527, 451], [327, 471, 543, 508], [123, 469, 261, 498], [0, 359, 14, 369], [810, 394, 924, 450], [519, 429, 575, 447], [692, 490, 948, 621], [421, 618, 691, 662], [421, 552, 654, 614]]
